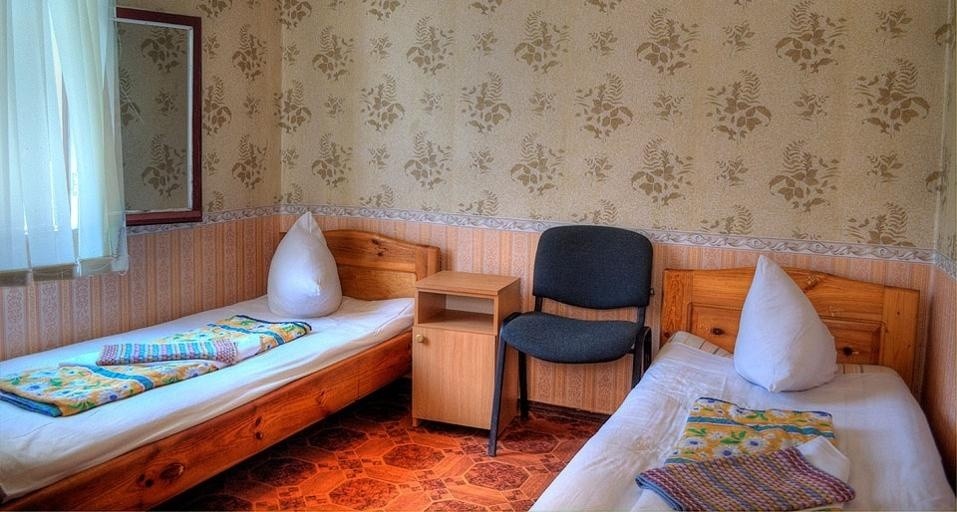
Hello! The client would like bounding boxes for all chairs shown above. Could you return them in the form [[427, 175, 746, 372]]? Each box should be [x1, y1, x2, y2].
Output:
[[487, 224, 657, 458]]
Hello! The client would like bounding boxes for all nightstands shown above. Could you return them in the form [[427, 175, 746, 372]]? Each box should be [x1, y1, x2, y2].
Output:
[[408, 270, 522, 436]]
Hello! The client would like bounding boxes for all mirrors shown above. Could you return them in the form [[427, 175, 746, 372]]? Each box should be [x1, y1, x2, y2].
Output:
[[111, 7, 204, 227]]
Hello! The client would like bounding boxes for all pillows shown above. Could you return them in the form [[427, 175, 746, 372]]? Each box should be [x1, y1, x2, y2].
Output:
[[267, 208, 351, 320], [728, 256, 839, 391]]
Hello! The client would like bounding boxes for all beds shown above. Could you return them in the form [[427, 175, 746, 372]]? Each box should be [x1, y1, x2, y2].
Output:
[[0, 229, 444, 512], [523, 265, 956, 512]]
